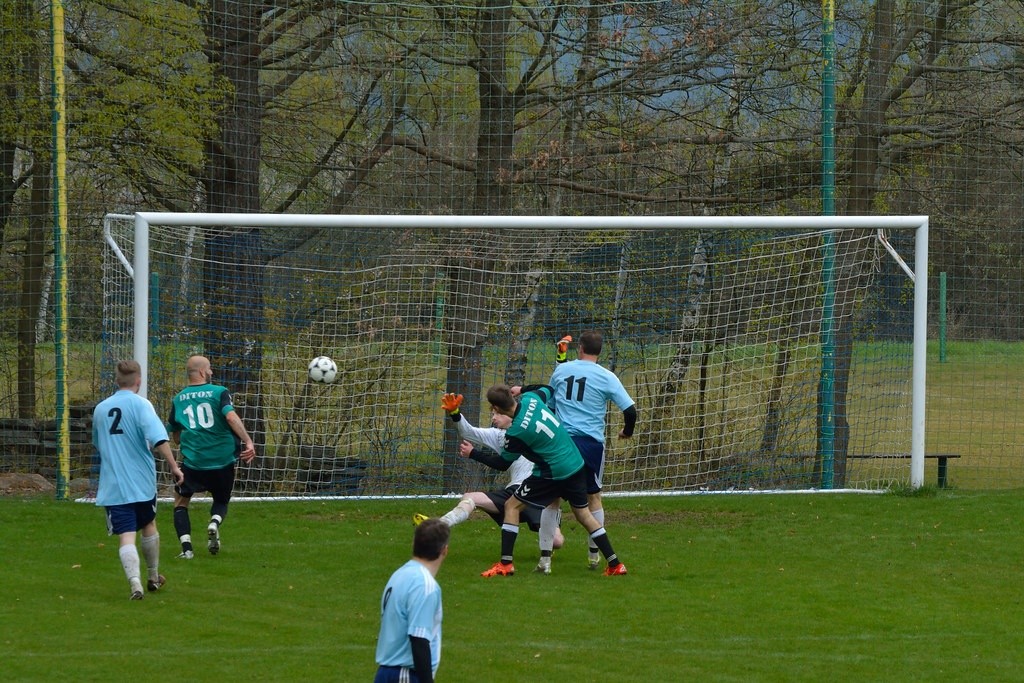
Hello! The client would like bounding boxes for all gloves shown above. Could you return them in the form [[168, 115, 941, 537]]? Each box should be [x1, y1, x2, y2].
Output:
[[557, 336, 572, 362], [441, 393, 463, 414]]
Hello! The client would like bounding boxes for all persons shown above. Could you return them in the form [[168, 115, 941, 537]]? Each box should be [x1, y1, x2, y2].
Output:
[[92, 360, 184, 601], [371, 519, 451, 683], [459, 383, 629, 576], [529, 330, 637, 576], [412, 335, 572, 549], [168, 355, 256, 559]]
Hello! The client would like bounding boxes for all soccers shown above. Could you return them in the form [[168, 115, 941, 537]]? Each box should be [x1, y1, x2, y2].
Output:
[[308, 355, 338, 384]]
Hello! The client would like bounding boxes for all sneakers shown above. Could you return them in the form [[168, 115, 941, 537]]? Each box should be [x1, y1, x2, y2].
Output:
[[588, 555, 600, 569], [481, 562, 514, 578], [175, 550, 193, 560], [601, 563, 627, 575], [533, 563, 551, 575], [413, 512, 428, 526], [129, 590, 143, 600], [207, 524, 220, 556], [147, 575, 166, 592]]
